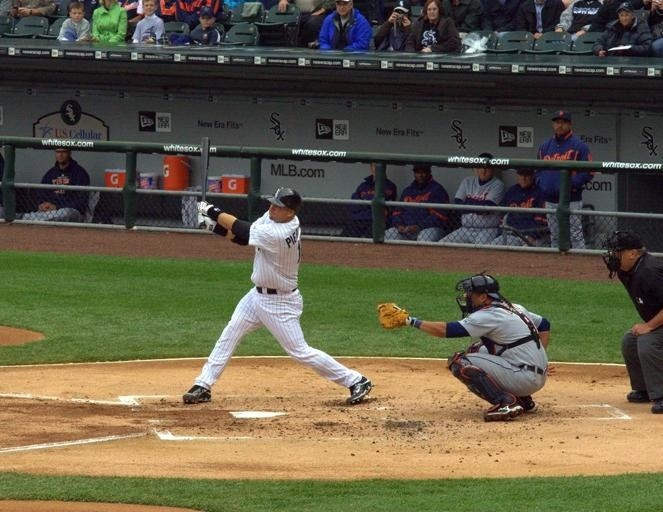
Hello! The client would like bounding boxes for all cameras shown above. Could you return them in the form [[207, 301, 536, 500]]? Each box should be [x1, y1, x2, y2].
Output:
[[398, 15, 404, 21]]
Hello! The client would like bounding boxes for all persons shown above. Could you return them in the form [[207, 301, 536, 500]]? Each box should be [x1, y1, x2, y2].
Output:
[[0, 153, 7, 218], [1, 1, 662, 57], [534, 109, 594, 248], [11, 148, 91, 222], [385, 163, 451, 242], [342, 162, 396, 238], [439, 152, 501, 247], [181, 186, 372, 405], [491, 168, 550, 247], [600, 227, 663, 415], [377, 271, 550, 420]]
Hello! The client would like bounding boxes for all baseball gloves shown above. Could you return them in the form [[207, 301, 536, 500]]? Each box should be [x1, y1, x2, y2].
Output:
[[377, 303, 410, 328]]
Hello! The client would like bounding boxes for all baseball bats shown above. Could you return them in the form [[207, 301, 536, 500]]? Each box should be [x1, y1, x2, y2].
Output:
[[201, 136, 210, 228]]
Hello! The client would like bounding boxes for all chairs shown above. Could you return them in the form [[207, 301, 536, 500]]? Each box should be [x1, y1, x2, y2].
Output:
[[1, 0, 661, 59]]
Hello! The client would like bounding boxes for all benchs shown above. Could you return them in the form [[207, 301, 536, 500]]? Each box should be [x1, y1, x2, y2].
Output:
[[1, 189, 598, 246]]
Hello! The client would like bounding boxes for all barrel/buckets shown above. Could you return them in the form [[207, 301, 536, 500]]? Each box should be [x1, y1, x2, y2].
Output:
[[207, 175, 222, 193], [162, 154, 192, 191], [138, 172, 162, 189]]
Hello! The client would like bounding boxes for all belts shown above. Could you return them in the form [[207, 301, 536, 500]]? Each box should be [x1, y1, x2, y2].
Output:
[[256, 286, 296, 295], [520, 363, 544, 375]]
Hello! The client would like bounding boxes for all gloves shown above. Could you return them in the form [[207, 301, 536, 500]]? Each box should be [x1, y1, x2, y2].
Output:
[[197, 200, 227, 237]]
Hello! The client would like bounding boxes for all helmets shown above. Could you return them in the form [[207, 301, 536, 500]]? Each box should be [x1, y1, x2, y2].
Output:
[[265, 187, 301, 209], [603, 230, 642, 272], [455, 275, 502, 313]]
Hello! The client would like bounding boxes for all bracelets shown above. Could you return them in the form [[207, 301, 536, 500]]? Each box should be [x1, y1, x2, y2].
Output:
[[414, 320, 422, 328], [207, 205, 221, 220], [212, 223, 228, 237]]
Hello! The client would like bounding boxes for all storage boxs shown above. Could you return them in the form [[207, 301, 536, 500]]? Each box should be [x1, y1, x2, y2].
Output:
[[102, 147, 257, 194]]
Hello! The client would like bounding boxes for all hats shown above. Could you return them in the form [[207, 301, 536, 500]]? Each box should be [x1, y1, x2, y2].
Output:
[[393, 0, 410, 14], [552, 111, 572, 122], [616, 2, 633, 13], [201, 6, 214, 16]]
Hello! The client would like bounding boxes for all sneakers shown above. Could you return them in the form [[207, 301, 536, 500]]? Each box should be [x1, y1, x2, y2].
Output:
[[628, 391, 663, 413], [184, 387, 211, 403], [485, 396, 538, 421], [347, 376, 372, 403]]
